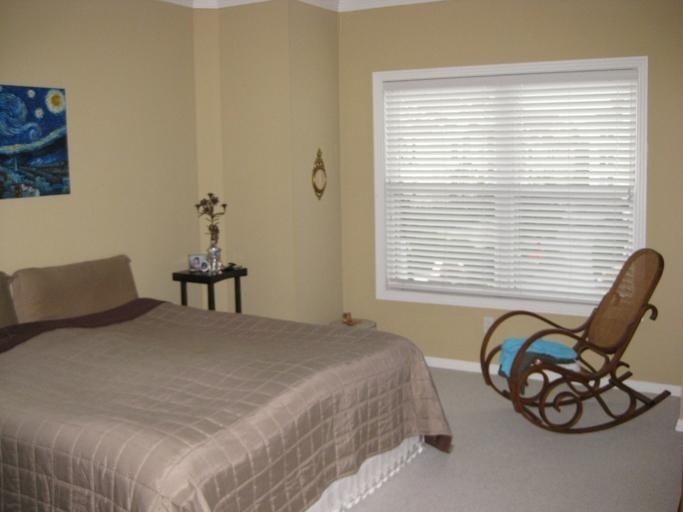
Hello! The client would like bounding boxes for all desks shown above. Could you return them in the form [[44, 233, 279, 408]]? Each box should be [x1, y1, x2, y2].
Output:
[[173, 268, 248, 314]]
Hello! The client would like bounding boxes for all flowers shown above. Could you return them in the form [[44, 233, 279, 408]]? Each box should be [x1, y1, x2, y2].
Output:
[[193, 193, 227, 239]]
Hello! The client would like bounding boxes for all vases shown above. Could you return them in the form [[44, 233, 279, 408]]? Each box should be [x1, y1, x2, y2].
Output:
[[208, 240, 221, 262]]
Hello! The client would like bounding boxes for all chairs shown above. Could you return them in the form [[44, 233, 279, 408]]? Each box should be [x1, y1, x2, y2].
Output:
[[480, 248, 671, 434]]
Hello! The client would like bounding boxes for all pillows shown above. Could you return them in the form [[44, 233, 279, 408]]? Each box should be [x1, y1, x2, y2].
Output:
[[11, 256, 137, 324], [0, 270, 17, 330]]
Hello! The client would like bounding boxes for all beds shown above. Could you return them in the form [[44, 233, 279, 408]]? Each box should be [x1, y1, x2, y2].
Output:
[[1, 299, 453, 511]]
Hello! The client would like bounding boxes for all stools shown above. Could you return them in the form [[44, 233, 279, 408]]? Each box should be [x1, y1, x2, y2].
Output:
[[330, 318, 376, 328]]
